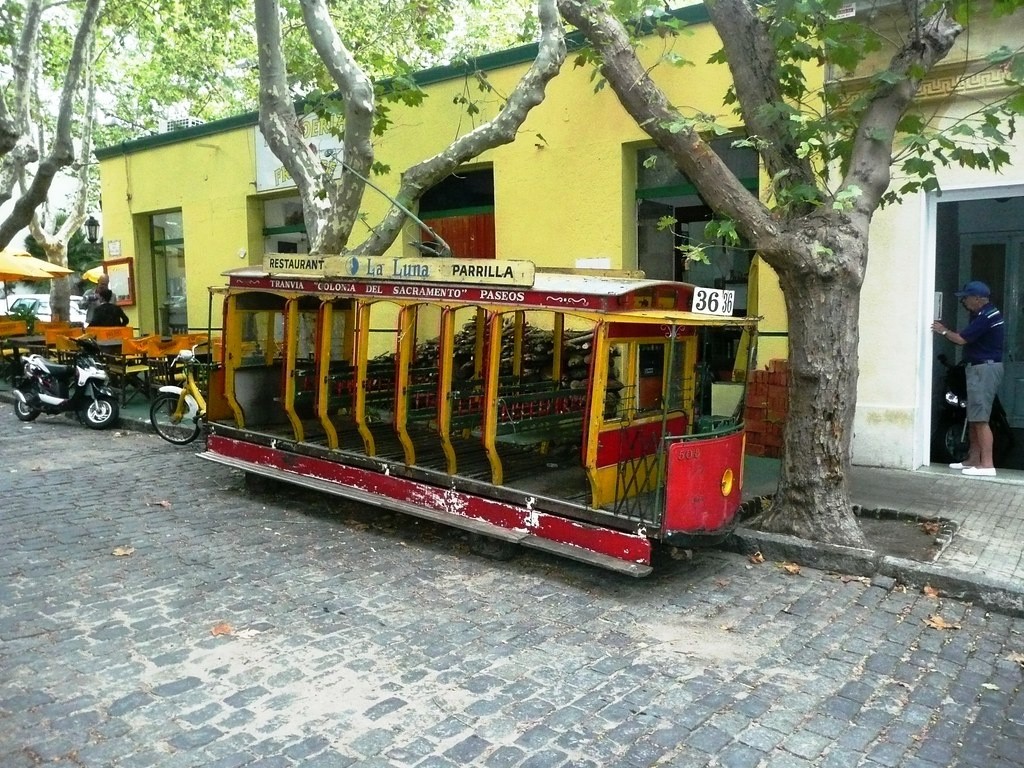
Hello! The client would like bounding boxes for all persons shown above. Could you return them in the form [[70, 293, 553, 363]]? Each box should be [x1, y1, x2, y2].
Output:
[[931, 282, 1005, 476], [78, 274, 116, 323], [90, 288, 129, 327]]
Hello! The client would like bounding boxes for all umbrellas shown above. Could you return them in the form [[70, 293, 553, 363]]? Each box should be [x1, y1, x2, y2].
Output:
[[0, 248, 75, 311]]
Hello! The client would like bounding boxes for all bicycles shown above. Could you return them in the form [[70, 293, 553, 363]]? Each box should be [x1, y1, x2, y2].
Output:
[[148, 341, 208, 443]]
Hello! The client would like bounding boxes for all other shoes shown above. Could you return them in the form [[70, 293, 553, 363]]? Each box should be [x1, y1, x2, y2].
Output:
[[948, 462, 975, 469], [961, 466, 997, 476]]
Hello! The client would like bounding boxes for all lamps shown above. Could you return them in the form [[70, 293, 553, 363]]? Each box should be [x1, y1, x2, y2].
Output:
[[84, 217, 103, 247]]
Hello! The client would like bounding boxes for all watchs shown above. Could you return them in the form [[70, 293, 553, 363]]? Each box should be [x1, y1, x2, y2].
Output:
[[942, 331, 947, 335]]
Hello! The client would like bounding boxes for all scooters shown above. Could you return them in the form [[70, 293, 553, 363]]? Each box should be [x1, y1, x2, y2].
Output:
[[937, 355, 976, 462], [12, 333, 120, 431]]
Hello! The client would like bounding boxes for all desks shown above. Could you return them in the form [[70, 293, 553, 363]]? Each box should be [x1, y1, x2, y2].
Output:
[[6, 336, 46, 389], [98, 336, 173, 385]]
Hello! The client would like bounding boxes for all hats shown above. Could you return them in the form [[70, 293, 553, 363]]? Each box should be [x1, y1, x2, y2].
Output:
[[954, 281, 990, 297]]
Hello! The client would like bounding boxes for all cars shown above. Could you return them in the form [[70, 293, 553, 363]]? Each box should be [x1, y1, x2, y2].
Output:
[[7, 294, 89, 331]]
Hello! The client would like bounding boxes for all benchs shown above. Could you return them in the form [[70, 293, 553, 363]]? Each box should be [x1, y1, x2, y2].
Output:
[[0, 317, 222, 413], [274, 363, 611, 470]]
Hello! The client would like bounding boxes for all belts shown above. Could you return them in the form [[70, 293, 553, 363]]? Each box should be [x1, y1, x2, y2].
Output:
[[964, 360, 1002, 367]]
